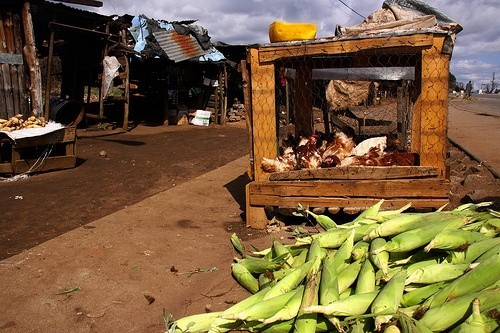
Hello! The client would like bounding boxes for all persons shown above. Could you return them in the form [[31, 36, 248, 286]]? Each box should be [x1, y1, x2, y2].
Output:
[[465, 81, 473, 96]]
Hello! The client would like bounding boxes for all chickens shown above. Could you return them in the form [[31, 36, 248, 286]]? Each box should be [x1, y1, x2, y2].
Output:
[[260, 125, 401, 174]]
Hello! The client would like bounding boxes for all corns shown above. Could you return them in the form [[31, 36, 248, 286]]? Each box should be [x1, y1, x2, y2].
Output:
[[161, 199, 500, 333], [0, 114, 48, 133]]
